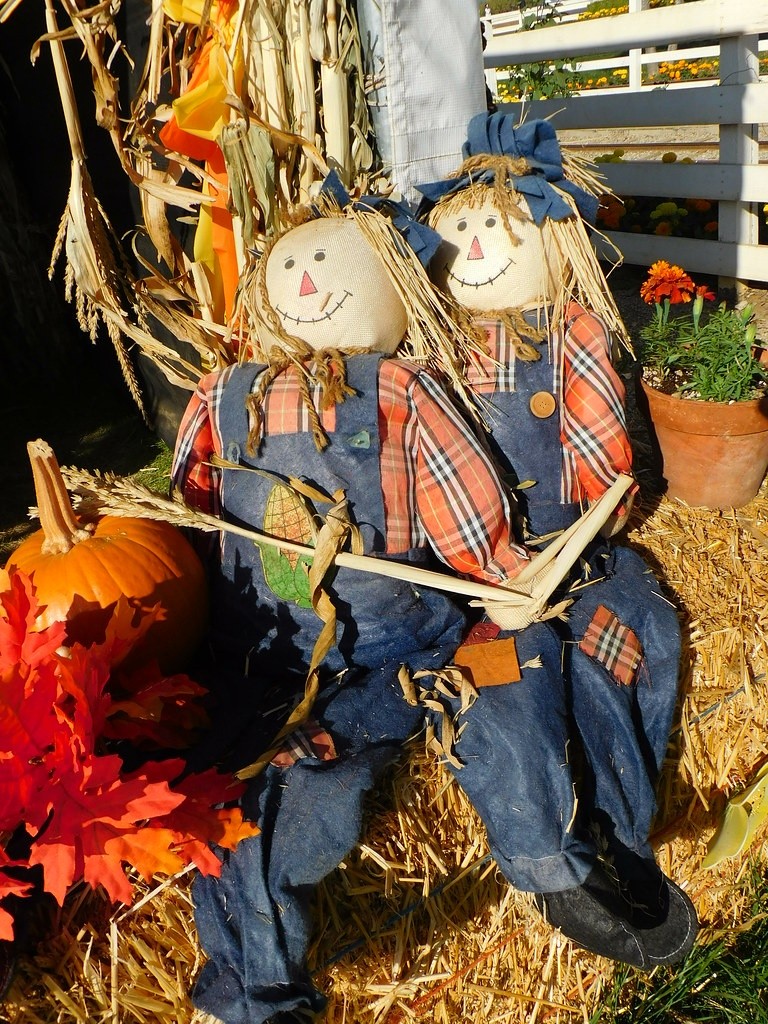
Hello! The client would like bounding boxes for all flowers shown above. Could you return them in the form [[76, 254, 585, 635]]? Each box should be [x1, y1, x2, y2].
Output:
[[637, 260, 768, 400]]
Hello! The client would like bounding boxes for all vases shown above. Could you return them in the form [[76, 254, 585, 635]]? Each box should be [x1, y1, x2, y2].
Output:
[[633, 346, 768, 511]]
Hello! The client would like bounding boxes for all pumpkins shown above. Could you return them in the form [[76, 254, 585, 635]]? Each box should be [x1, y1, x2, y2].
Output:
[[5, 439, 205, 668]]
